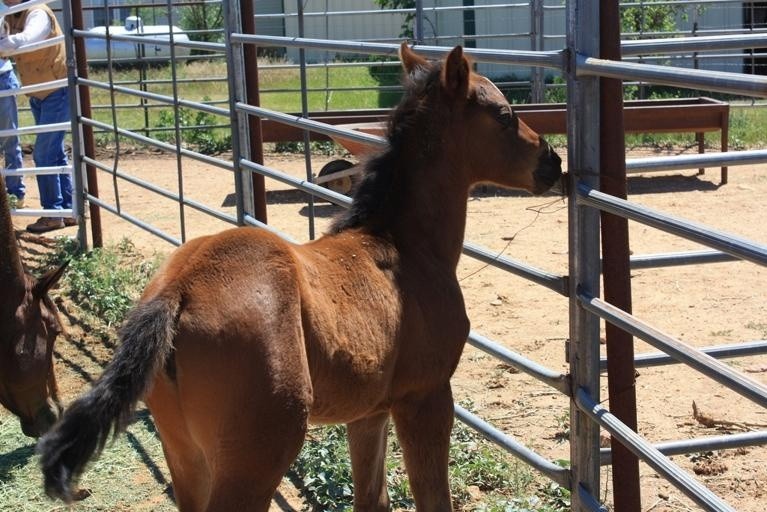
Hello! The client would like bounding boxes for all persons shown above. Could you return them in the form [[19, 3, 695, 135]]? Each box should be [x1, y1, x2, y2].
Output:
[[0, 0, 78, 233], [0, 57, 26, 209]]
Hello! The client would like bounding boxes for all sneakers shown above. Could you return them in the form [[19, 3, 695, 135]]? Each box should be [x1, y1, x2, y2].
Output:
[[17, 199, 26, 209], [26, 214, 77, 234]]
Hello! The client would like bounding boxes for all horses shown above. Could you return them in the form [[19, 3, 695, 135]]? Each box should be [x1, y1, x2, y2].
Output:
[[34, 38, 563, 512], [1, 163, 73, 439]]
